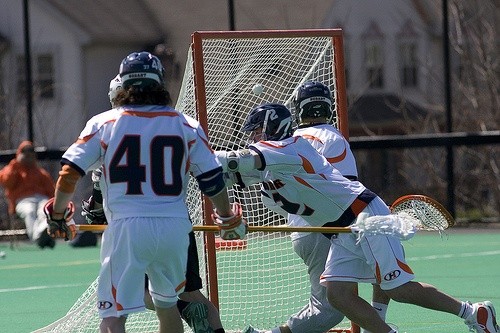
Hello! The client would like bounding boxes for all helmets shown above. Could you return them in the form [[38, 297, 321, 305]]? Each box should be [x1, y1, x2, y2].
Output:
[[108, 73, 123, 108], [119, 51, 167, 89], [291, 80, 333, 121], [240, 103, 292, 142]]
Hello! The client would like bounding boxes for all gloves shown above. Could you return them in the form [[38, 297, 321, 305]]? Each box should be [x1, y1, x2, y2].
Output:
[[43, 197, 77, 241], [210, 201, 248, 240], [79, 195, 109, 225]]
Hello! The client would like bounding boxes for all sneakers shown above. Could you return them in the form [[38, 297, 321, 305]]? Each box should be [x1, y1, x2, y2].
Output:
[[241, 324, 256, 333], [463, 301, 500, 333]]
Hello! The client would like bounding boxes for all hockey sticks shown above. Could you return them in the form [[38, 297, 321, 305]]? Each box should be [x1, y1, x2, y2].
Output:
[[63, 194, 456, 241]]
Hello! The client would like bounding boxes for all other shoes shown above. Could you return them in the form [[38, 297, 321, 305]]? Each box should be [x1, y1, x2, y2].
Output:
[[176, 299, 209, 333]]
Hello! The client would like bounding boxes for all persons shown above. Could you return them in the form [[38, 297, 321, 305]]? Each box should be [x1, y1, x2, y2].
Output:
[[213, 104, 500, 333], [241, 79, 405, 333], [44, 52, 248, 333], [0, 141, 56, 250], [81, 166, 225, 333]]
